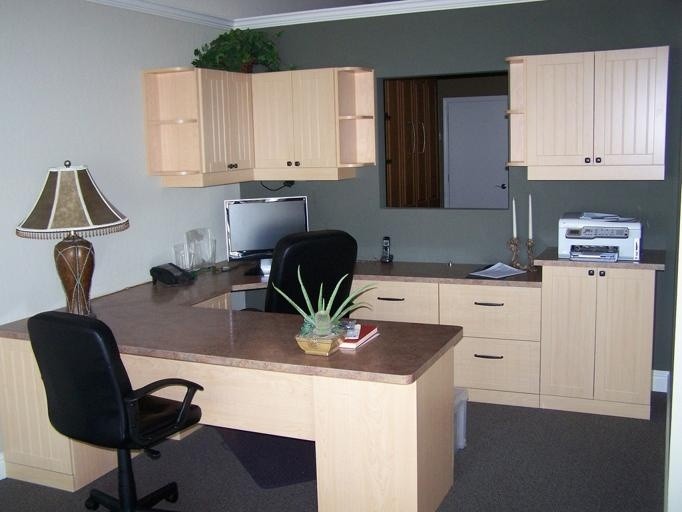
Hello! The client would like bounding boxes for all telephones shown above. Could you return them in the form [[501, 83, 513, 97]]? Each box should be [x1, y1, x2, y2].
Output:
[[380, 236, 394, 263], [150, 262, 195, 285]]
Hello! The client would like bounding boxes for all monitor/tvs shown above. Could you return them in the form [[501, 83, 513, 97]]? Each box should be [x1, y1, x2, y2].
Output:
[[223, 195, 310, 276]]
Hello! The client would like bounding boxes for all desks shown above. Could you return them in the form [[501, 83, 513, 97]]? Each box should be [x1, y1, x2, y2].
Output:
[[0, 305, 463, 512]]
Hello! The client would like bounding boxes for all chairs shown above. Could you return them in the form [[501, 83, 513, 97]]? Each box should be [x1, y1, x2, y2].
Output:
[[241, 229, 358, 318], [27, 311, 203, 511]]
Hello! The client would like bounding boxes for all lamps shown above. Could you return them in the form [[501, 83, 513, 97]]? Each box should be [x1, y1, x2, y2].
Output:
[[16, 160, 129, 319]]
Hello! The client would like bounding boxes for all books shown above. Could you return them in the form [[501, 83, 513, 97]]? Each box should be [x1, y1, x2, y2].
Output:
[[339, 322, 377, 349], [339, 334, 379, 354]]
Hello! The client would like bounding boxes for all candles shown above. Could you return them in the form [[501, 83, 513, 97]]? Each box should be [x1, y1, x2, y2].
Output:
[[529, 192, 533, 240], [512, 196, 516, 238]]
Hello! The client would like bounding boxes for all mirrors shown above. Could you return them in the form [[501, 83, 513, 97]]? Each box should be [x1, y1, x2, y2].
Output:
[[382, 71, 509, 210]]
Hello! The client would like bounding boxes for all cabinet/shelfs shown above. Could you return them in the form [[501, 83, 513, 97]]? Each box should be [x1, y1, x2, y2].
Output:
[[384, 78, 440, 208], [438, 282, 542, 410], [506, 55, 528, 168], [523, 45, 669, 180], [337, 65, 377, 168], [198, 66, 252, 188], [252, 66, 336, 181], [348, 280, 439, 325], [539, 265, 656, 421], [145, 67, 201, 178], [195, 292, 231, 310]]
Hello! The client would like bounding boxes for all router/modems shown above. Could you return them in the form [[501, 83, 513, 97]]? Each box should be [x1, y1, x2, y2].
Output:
[[208, 239, 240, 271]]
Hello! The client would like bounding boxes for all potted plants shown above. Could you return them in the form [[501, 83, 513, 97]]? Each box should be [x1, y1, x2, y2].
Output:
[[272, 263, 378, 356], [191, 28, 297, 73]]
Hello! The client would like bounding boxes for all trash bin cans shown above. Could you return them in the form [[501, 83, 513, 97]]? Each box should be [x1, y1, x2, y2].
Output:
[[453, 390, 468, 452]]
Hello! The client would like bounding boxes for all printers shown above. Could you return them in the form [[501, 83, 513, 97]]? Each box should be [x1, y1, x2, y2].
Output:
[[558, 212, 643, 262]]
